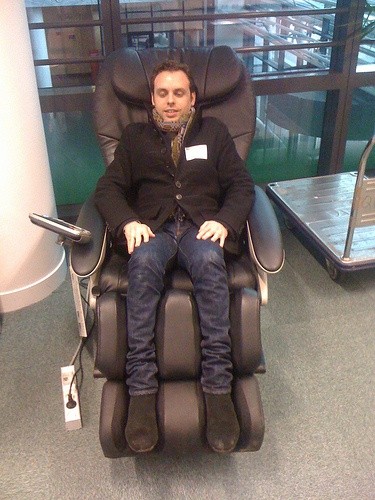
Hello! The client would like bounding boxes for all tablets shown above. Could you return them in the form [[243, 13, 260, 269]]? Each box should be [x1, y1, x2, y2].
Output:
[[29, 213, 88, 240]]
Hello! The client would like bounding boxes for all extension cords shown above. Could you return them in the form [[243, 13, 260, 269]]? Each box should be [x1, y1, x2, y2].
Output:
[[60, 365, 83, 431]]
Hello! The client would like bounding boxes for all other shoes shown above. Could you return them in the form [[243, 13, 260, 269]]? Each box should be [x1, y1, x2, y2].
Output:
[[125, 393, 158, 452], [205, 392, 240, 453]]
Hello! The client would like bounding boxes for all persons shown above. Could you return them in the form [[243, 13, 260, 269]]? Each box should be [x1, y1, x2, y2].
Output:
[[90, 59, 258, 455]]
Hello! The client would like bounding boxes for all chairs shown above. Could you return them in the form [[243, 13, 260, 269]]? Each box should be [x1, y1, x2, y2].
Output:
[[68, 45, 286, 459]]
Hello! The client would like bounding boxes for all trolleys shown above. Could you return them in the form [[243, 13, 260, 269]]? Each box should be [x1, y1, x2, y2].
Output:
[[264, 135, 375, 284]]
